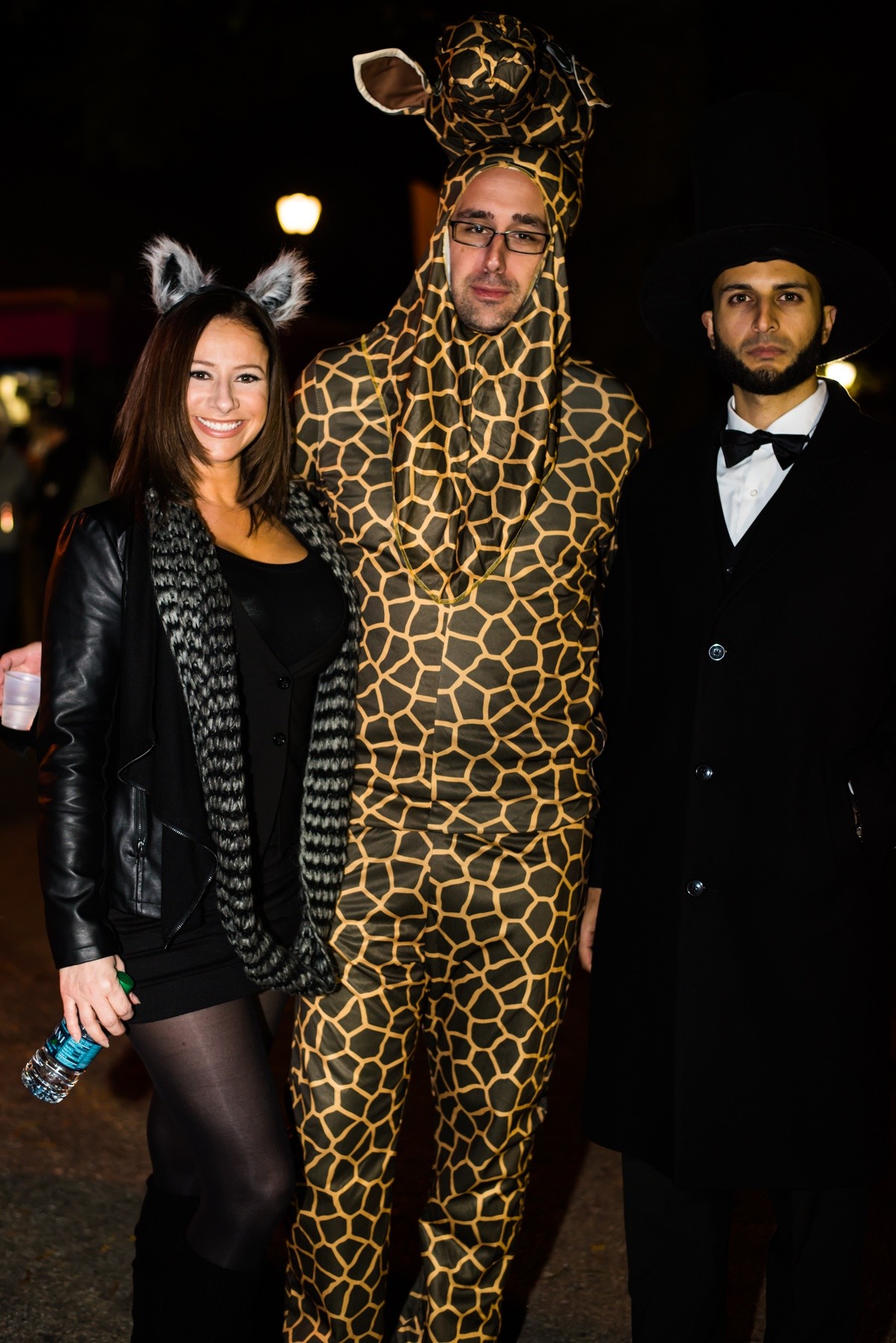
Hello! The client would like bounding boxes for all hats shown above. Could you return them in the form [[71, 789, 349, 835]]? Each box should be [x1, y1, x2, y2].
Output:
[[623, 85, 892, 373]]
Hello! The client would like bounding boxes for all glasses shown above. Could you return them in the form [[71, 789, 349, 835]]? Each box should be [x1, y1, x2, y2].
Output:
[[448, 217, 552, 255]]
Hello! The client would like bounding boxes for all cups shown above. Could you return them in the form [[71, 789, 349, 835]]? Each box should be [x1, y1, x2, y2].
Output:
[[2, 671, 40, 730]]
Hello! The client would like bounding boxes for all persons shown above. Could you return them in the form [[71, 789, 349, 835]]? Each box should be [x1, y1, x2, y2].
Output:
[[3, 144, 649, 1342], [24, 281, 364, 1343], [568, 205, 896, 1342]]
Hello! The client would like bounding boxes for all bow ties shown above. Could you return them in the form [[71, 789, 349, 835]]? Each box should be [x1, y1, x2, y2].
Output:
[[717, 427, 810, 472]]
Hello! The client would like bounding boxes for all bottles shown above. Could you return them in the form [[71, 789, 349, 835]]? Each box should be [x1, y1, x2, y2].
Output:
[[21, 971, 134, 1103]]
